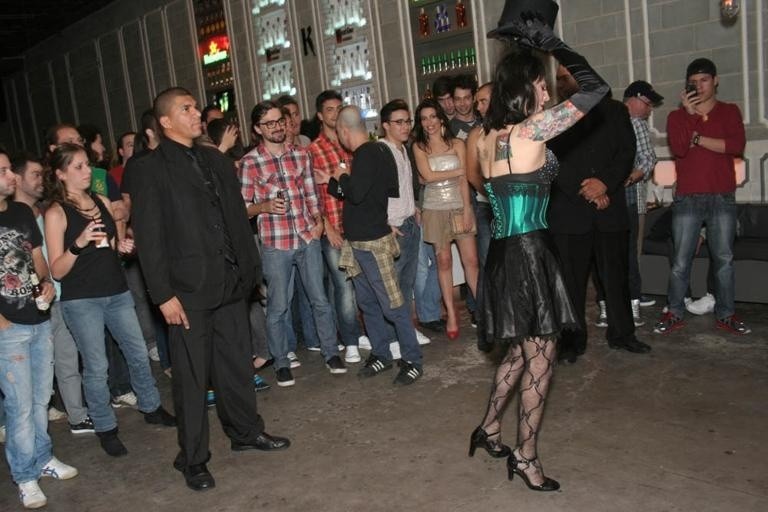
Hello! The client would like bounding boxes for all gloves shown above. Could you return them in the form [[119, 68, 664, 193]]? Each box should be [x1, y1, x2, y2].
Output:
[[507, 10, 611, 116]]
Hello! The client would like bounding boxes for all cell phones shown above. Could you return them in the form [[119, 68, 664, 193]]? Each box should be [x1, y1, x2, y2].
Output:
[[685, 80, 697, 100]]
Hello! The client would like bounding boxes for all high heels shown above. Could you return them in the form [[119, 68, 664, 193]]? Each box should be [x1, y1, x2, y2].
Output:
[[467, 426, 513, 458], [445, 308, 461, 340], [506, 452, 558, 491]]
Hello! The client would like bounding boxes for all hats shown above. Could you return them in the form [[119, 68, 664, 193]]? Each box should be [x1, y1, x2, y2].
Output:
[[624, 78, 666, 104], [685, 58, 719, 88], [485, 0, 556, 52]]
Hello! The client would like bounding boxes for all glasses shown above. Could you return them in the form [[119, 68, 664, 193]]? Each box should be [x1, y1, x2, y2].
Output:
[[258, 115, 286, 129], [391, 117, 414, 125]]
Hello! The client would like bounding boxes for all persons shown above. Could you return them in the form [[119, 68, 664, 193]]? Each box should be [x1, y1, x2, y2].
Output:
[[466, 20, 612, 490], [0, 73, 492, 508], [545, 57, 751, 361]]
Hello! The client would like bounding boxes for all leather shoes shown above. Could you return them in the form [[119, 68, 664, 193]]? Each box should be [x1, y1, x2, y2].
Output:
[[226, 432, 291, 451], [186, 469, 213, 491], [254, 355, 275, 371], [558, 333, 587, 362], [606, 331, 652, 355], [422, 316, 445, 333]]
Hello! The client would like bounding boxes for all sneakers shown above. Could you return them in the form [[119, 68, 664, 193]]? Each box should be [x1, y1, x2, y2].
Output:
[[68, 419, 129, 458], [357, 354, 391, 378], [393, 362, 425, 386], [595, 301, 608, 329], [110, 393, 179, 427], [206, 386, 216, 408], [276, 349, 301, 387], [414, 329, 432, 347], [41, 457, 78, 480], [253, 375, 271, 392], [16, 479, 48, 510], [308, 334, 372, 375], [685, 292, 718, 316], [49, 408, 67, 421], [651, 310, 687, 335], [713, 314, 753, 335], [630, 294, 657, 327], [389, 338, 402, 360]]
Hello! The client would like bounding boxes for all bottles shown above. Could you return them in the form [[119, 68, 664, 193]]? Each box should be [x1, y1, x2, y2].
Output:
[[248, 17, 297, 103], [335, 158, 348, 201], [326, 0, 374, 115], [27, 266, 52, 317], [415, 0, 480, 104]]
